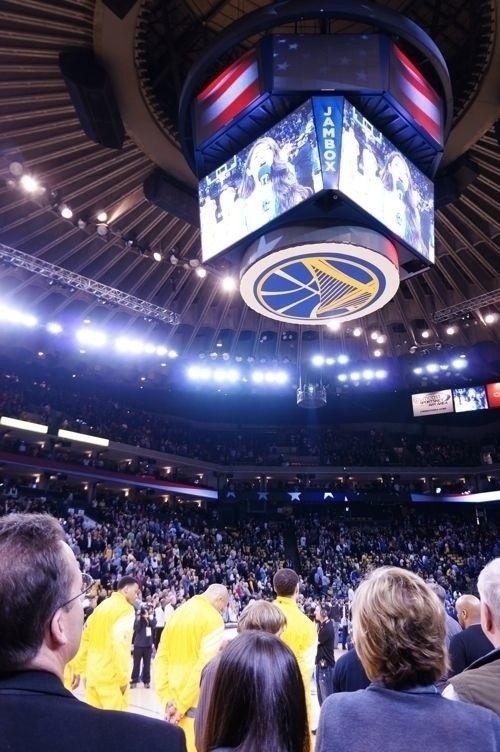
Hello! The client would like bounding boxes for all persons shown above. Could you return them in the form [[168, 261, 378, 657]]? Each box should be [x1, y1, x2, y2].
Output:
[[341, 106, 434, 261], [63, 557, 499, 752], [0, 370, 500, 557], [199, 102, 315, 239], [1, 513, 188, 752]]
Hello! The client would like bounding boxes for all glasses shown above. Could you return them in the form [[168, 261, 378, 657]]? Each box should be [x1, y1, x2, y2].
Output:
[[52, 574, 94, 620]]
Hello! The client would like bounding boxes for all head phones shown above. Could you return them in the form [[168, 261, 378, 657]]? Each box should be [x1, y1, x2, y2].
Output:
[[320, 602, 327, 617]]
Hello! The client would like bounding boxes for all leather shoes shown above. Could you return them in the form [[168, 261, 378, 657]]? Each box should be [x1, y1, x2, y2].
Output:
[[130, 680, 150, 688]]
